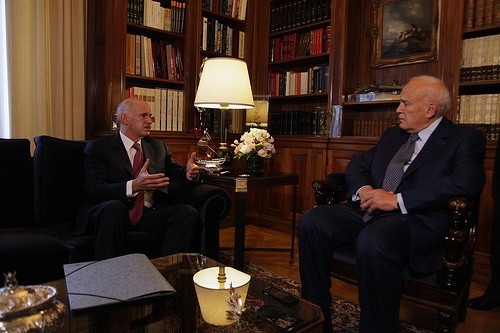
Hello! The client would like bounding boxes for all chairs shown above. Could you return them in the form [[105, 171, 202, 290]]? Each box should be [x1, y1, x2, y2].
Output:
[[312, 125, 480, 333]]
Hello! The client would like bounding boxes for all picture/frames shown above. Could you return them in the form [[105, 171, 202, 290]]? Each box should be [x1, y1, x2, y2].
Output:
[[369, 0, 441, 69]]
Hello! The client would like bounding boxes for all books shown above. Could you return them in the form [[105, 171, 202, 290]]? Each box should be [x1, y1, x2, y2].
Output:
[[127, 0, 186, 33], [271, 0, 331, 32], [464, 0, 500, 29], [460, 34, 500, 81], [273, 25, 331, 62], [270, 107, 327, 135], [127, 87, 183, 131], [201, 17, 245, 59], [272, 63, 329, 96], [456, 93, 500, 139], [126, 34, 184, 81], [202, 0, 247, 20]]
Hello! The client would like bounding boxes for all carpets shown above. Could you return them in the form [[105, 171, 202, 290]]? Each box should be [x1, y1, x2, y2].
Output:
[[219, 250, 434, 333]]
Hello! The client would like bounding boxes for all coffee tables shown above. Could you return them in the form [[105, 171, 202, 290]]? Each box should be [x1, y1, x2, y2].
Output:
[[0, 254, 325, 333], [200, 171, 300, 271]]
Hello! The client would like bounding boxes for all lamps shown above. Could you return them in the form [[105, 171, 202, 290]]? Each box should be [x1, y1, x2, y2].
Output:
[[194, 57, 255, 168], [246, 100, 268, 128], [192, 262, 252, 325]]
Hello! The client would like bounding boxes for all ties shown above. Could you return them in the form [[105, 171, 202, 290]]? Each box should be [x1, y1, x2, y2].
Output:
[[128, 143, 144, 226], [363, 132, 420, 223]]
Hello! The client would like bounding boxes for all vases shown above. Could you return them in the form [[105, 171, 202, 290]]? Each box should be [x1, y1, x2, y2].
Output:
[[245, 154, 264, 178]]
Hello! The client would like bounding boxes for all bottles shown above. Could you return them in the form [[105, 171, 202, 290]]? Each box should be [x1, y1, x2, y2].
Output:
[[218, 142, 228, 158]]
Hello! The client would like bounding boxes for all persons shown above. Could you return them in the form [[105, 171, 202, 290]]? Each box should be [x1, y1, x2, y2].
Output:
[[297, 75, 484, 333], [468, 138, 500, 309], [73, 97, 199, 262]]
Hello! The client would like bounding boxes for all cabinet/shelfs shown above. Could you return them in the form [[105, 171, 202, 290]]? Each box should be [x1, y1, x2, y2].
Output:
[[87, 0, 500, 285]]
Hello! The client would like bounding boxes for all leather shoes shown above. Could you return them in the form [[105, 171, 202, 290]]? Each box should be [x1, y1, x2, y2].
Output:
[[468, 277, 500, 310]]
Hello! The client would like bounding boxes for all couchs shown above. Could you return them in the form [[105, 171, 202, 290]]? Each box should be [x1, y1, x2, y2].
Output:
[[0, 136, 232, 287]]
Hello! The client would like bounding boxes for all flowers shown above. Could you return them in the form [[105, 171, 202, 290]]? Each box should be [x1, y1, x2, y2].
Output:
[[233, 127, 275, 158]]
